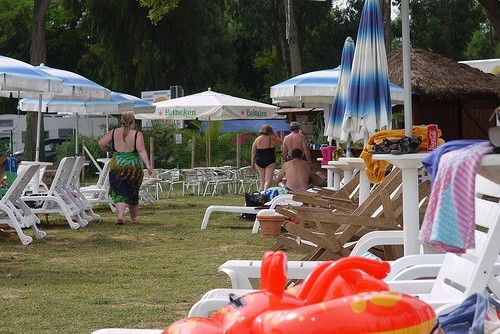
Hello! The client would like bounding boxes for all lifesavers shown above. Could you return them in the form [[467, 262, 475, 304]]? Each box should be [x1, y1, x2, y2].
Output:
[[158, 250, 437, 334]]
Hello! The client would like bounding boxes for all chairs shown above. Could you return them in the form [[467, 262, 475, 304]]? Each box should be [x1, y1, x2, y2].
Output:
[[0, 113, 500, 334]]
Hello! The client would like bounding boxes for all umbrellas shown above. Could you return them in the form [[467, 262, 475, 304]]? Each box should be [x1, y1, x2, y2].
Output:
[[0, 1, 392, 206], [386, 44, 500, 95]]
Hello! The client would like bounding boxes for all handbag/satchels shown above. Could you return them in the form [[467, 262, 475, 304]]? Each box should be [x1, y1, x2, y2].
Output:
[[242, 191, 265, 221]]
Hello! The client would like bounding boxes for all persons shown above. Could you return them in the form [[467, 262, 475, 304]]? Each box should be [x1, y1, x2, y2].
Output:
[[275, 148, 314, 193], [97, 111, 153, 224], [282, 121, 312, 162], [251, 123, 282, 191]]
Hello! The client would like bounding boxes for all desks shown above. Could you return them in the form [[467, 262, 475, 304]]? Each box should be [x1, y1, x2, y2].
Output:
[[328, 161, 354, 184], [372, 152, 430, 255], [321, 165, 343, 190], [339, 158, 370, 206]]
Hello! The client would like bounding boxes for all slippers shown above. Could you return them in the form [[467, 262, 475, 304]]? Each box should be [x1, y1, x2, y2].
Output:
[[116, 221, 123, 224]]
